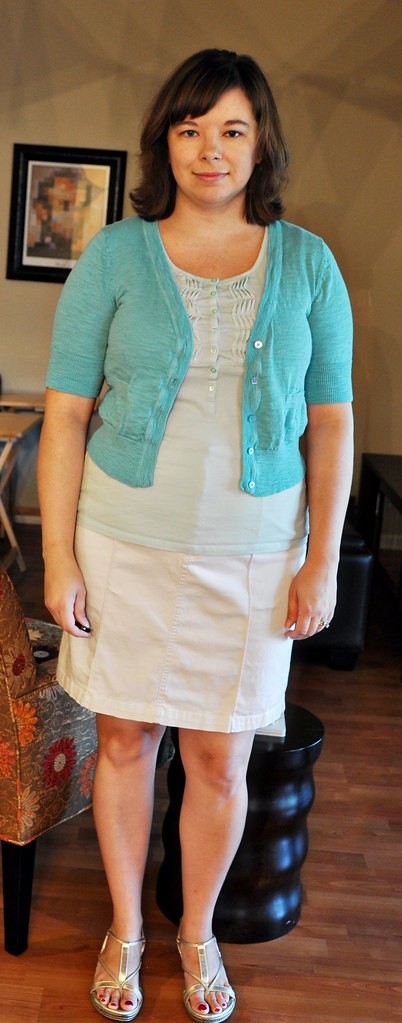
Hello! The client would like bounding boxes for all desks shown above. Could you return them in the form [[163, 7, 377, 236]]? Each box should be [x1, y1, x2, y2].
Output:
[[321, 505, 377, 672]]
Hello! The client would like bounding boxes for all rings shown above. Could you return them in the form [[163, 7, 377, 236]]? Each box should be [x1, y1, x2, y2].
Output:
[[320, 621, 329, 628]]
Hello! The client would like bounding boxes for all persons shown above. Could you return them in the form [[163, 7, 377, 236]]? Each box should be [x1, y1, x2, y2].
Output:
[[40, 48, 354, 1023]]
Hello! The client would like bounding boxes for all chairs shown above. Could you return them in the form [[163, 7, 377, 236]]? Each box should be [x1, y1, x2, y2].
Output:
[[0, 410, 46, 572]]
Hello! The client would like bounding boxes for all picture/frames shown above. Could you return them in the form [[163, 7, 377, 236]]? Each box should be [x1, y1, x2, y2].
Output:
[[6, 142, 127, 285]]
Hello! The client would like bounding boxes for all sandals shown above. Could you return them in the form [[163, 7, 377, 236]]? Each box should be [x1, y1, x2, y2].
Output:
[[90, 924, 146, 1021], [175, 924, 236, 1023]]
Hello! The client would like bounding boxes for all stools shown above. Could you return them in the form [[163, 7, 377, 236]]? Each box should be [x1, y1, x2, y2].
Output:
[[155, 702, 325, 944]]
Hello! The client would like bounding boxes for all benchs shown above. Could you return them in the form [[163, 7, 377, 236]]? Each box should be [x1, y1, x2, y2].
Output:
[[0, 566, 101, 955]]
